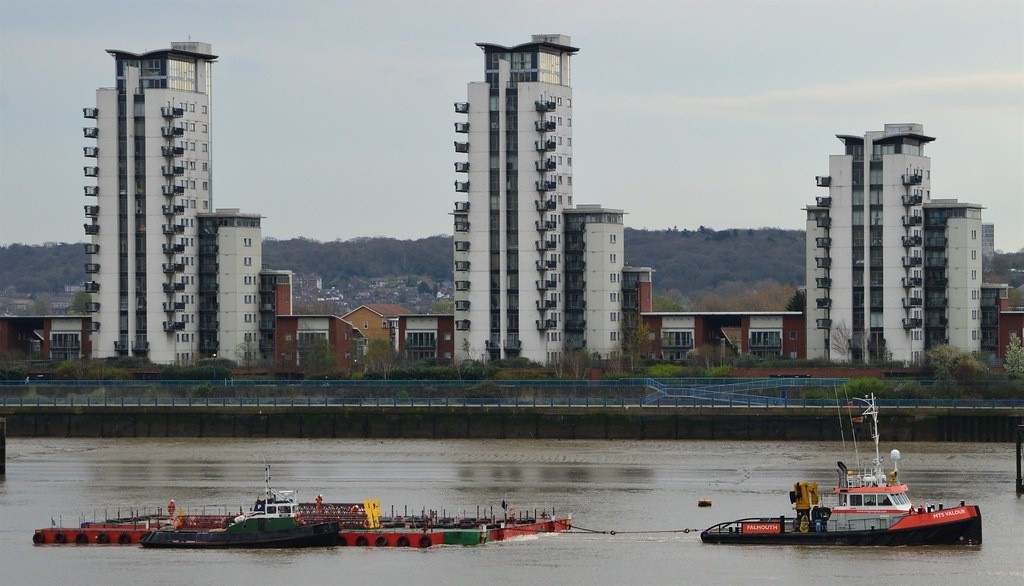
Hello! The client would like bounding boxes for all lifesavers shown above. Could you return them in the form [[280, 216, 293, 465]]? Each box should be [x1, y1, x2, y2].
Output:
[[172, 518, 180, 528], [419, 535, 432, 548], [32, 532, 46, 544], [396, 536, 410, 547], [351, 505, 360, 514], [81, 522, 89, 528], [75, 533, 88, 544], [811, 505, 832, 521], [295, 512, 300, 522], [363, 519, 371, 528], [118, 533, 131, 545], [338, 536, 347, 546], [97, 533, 110, 544], [374, 535, 388, 546], [54, 532, 66, 544], [508, 508, 516, 516], [355, 535, 369, 546]]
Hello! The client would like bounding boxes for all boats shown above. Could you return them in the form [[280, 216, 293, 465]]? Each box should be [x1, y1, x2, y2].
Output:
[[700, 380, 982, 545], [135, 450, 343, 549]]
